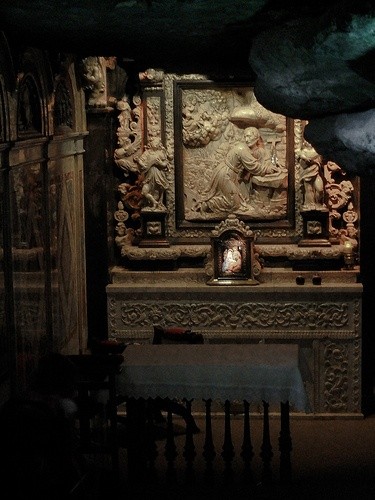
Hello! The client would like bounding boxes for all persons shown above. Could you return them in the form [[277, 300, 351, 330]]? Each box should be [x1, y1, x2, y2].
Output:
[[82, 55, 327, 277]]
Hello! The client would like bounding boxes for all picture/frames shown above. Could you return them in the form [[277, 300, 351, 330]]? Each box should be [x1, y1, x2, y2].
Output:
[[300, 210, 330, 246], [138, 212, 169, 247], [213, 237, 252, 280]]
